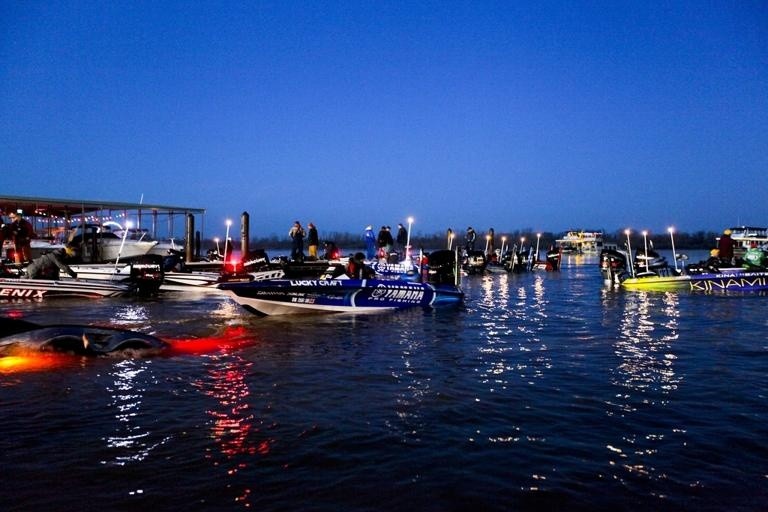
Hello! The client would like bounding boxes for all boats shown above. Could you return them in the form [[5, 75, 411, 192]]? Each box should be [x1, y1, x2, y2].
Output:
[[621, 268, 768, 293], [216, 249, 470, 316], [460, 249, 560, 275], [599, 249, 668, 272], [554, 231, 604, 254], [0, 231, 287, 299], [716, 224, 768, 256]]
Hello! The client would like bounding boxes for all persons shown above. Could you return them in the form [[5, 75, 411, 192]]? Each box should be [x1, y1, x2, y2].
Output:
[[289, 220, 307, 260], [396, 224, 408, 260], [364, 225, 375, 261], [322, 239, 337, 258], [22, 241, 79, 280], [385, 226, 393, 252], [304, 223, 319, 257], [9, 211, 36, 264], [351, 253, 376, 278], [377, 226, 387, 257]]
